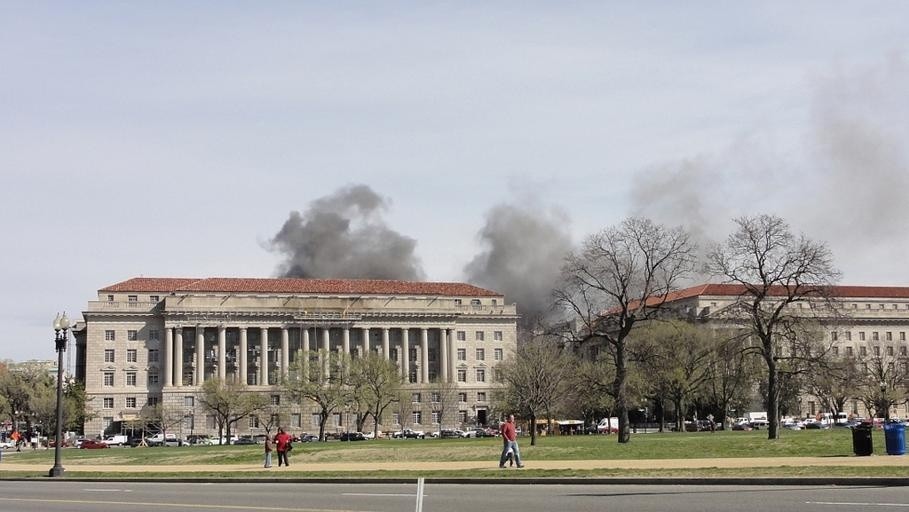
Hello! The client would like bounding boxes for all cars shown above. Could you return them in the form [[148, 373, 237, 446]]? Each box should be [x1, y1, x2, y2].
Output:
[[292, 432, 319, 442], [48, 435, 128, 449], [584, 425, 597, 435], [0, 441, 13, 449], [144, 434, 259, 447], [729, 411, 903, 431], [430, 427, 499, 438], [340, 431, 382, 441], [392, 429, 425, 439]]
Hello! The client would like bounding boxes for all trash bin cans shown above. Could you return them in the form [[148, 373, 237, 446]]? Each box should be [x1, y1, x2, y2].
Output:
[[852, 424, 906, 456]]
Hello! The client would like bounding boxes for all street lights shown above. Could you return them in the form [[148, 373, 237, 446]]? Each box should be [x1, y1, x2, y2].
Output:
[[49, 310, 70, 476]]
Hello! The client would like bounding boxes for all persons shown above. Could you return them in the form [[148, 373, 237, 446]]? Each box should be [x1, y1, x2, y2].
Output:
[[263, 425, 294, 468], [498, 413, 524, 470], [815, 410, 824, 429], [693, 413, 716, 432]]
